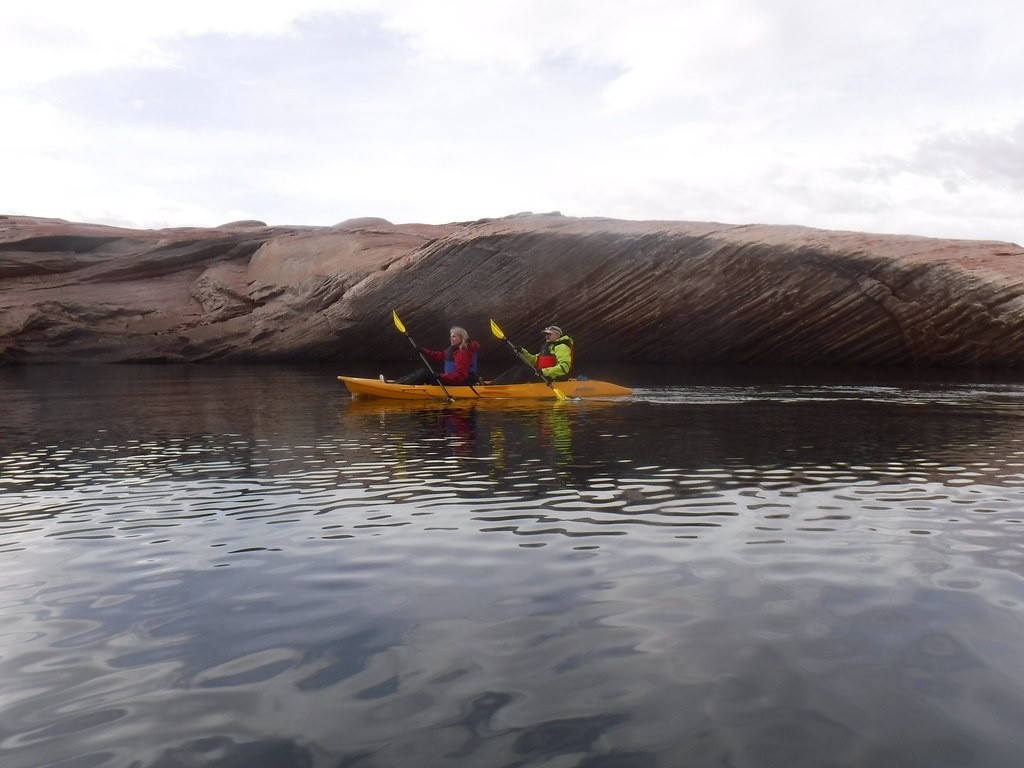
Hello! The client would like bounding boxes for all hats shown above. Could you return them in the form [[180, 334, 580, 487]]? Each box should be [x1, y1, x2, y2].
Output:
[[540, 326, 562, 335]]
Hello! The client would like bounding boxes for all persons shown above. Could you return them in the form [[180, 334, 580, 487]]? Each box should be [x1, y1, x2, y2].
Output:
[[379, 326, 480, 386], [476, 325, 574, 384]]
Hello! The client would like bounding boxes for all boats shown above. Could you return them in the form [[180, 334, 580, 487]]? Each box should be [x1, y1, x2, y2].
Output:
[[337, 374, 635, 401]]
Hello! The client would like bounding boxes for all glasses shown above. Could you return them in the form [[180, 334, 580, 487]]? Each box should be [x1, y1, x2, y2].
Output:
[[545, 327, 561, 334]]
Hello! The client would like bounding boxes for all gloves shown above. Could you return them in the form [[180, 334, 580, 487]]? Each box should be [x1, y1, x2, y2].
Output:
[[513, 344, 522, 355], [535, 369, 543, 377], [432, 373, 439, 381], [415, 347, 423, 353]]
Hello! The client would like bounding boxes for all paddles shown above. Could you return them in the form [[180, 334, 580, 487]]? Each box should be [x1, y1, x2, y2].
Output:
[[392, 309, 456, 402], [490, 319, 569, 401]]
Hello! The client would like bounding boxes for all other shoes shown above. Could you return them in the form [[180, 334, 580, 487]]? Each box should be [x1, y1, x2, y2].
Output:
[[379, 375, 388, 383]]
